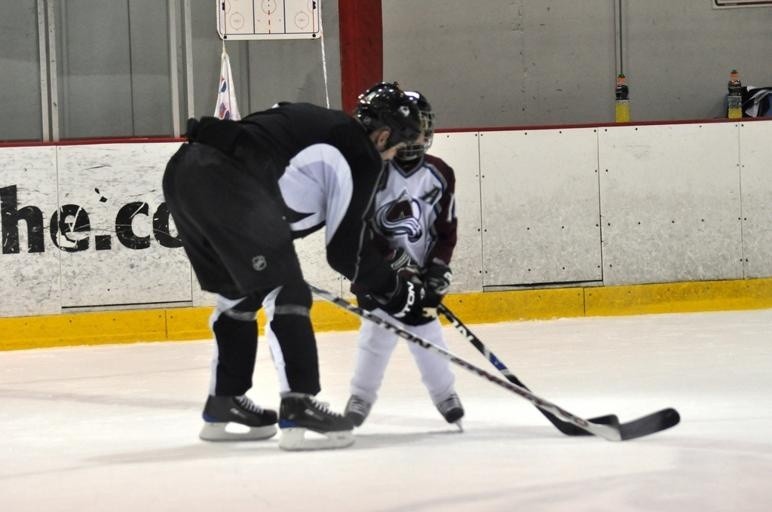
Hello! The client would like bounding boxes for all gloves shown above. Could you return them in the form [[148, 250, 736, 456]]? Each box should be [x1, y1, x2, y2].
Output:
[[389, 249, 421, 282], [427, 260, 454, 298], [387, 285, 439, 325]]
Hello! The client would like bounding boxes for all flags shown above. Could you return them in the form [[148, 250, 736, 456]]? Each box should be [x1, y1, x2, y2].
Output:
[[213, 52, 242, 122]]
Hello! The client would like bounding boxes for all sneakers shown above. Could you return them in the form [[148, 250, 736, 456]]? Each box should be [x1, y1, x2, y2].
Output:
[[203, 395, 277, 426], [346, 395, 370, 426], [435, 393, 463, 422], [280, 392, 353, 430]]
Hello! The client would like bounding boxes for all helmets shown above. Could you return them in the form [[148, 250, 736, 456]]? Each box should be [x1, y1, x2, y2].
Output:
[[358, 82, 419, 143], [396, 91, 435, 160]]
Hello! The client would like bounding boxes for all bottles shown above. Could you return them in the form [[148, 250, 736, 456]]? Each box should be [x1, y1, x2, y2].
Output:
[[727, 70, 742, 121], [614, 73, 630, 125]]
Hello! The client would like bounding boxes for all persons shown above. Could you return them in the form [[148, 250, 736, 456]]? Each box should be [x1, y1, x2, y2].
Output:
[[343, 88, 465, 427], [163, 81, 440, 432]]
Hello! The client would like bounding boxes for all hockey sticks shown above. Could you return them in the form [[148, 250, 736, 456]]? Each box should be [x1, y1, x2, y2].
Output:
[[305, 280, 681, 441], [420, 272, 620, 436]]
